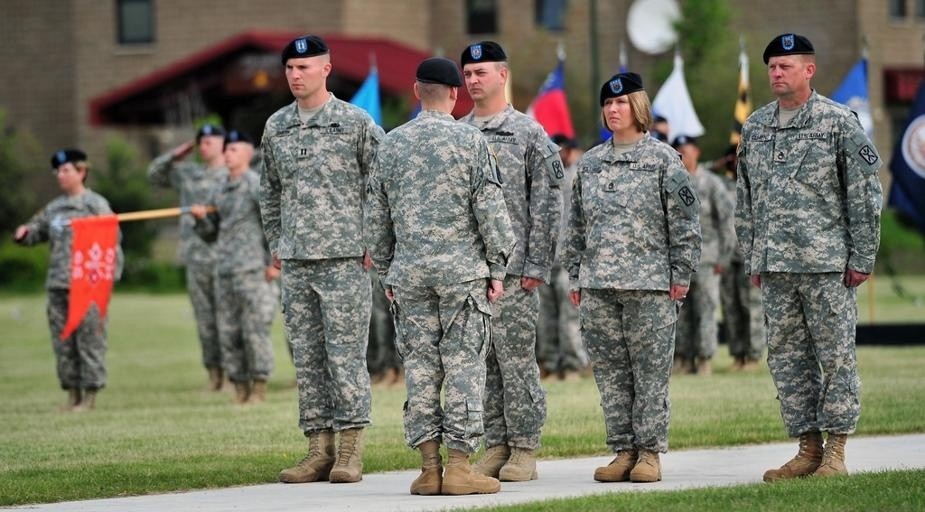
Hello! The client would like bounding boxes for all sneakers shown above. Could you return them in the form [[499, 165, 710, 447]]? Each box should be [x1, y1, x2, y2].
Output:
[[473, 445, 510, 478], [629, 448, 661, 482], [594, 450, 636, 480], [499, 445, 538, 481]]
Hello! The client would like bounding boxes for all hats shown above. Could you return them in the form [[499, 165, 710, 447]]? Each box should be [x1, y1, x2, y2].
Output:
[[461, 41, 506, 69], [650, 129, 667, 141], [281, 35, 328, 66], [671, 135, 695, 148], [225, 130, 254, 144], [52, 150, 86, 169], [551, 135, 567, 145], [763, 34, 816, 65], [197, 126, 226, 139], [416, 58, 464, 87], [600, 73, 642, 108]]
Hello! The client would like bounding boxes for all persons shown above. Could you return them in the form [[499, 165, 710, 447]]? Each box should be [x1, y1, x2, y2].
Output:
[[736, 32, 883, 482], [539, 134, 589, 387], [366, 284, 400, 388], [723, 146, 768, 365], [12, 147, 124, 416], [258, 34, 387, 483], [561, 74, 703, 482], [194, 129, 279, 405], [669, 135, 736, 374], [365, 57, 517, 495], [146, 125, 228, 391], [453, 42, 564, 480]]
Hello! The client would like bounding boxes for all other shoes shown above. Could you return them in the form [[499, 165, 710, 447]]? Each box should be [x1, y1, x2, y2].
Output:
[[728, 359, 759, 374], [380, 366, 406, 386], [676, 360, 709, 379], [544, 365, 583, 386]]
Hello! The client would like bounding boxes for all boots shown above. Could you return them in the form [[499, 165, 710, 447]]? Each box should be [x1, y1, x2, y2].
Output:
[[61, 393, 104, 411], [811, 432, 848, 475], [282, 433, 337, 483], [410, 440, 443, 493], [442, 448, 501, 493], [330, 431, 362, 484], [190, 367, 296, 405], [765, 432, 824, 482]]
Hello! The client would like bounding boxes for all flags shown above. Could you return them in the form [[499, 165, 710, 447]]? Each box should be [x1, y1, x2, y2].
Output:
[[524, 59, 573, 140], [651, 58, 705, 138], [731, 53, 752, 147], [60, 216, 118, 341], [827, 48, 873, 137], [888, 81, 924, 240], [350, 71, 382, 125]]
